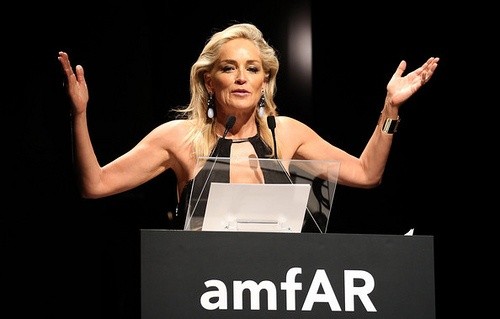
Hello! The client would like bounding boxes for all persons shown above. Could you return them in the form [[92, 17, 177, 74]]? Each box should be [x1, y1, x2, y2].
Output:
[[58, 22, 440, 227]]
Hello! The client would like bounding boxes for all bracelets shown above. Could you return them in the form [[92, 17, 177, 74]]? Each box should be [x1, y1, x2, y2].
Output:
[[377, 111, 401, 134]]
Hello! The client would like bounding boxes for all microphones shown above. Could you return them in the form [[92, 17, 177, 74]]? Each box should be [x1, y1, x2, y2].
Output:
[[215, 115, 236, 157], [266, 115, 277, 158]]
